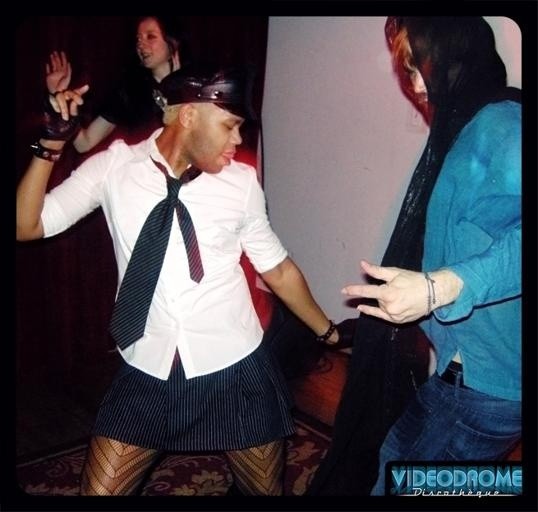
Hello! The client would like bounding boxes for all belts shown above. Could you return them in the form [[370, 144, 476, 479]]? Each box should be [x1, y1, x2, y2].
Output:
[[440, 368, 464, 388]]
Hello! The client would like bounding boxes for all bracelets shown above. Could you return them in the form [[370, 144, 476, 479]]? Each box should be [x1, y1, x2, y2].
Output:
[[313, 319, 335, 342], [424, 272, 436, 318], [28, 138, 64, 162]]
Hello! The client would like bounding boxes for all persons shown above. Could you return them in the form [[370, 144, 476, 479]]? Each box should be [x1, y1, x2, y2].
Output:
[[46, 13, 198, 153], [342, 15, 522, 497], [17, 65, 360, 496]]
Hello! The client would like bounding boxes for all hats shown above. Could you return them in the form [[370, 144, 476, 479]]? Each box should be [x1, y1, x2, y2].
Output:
[[156, 57, 261, 123]]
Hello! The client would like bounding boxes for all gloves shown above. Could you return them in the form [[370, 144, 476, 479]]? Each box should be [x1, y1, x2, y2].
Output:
[[40, 88, 83, 142]]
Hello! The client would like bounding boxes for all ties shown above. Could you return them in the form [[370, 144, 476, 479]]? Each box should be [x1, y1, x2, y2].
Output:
[[107, 163, 204, 351]]
